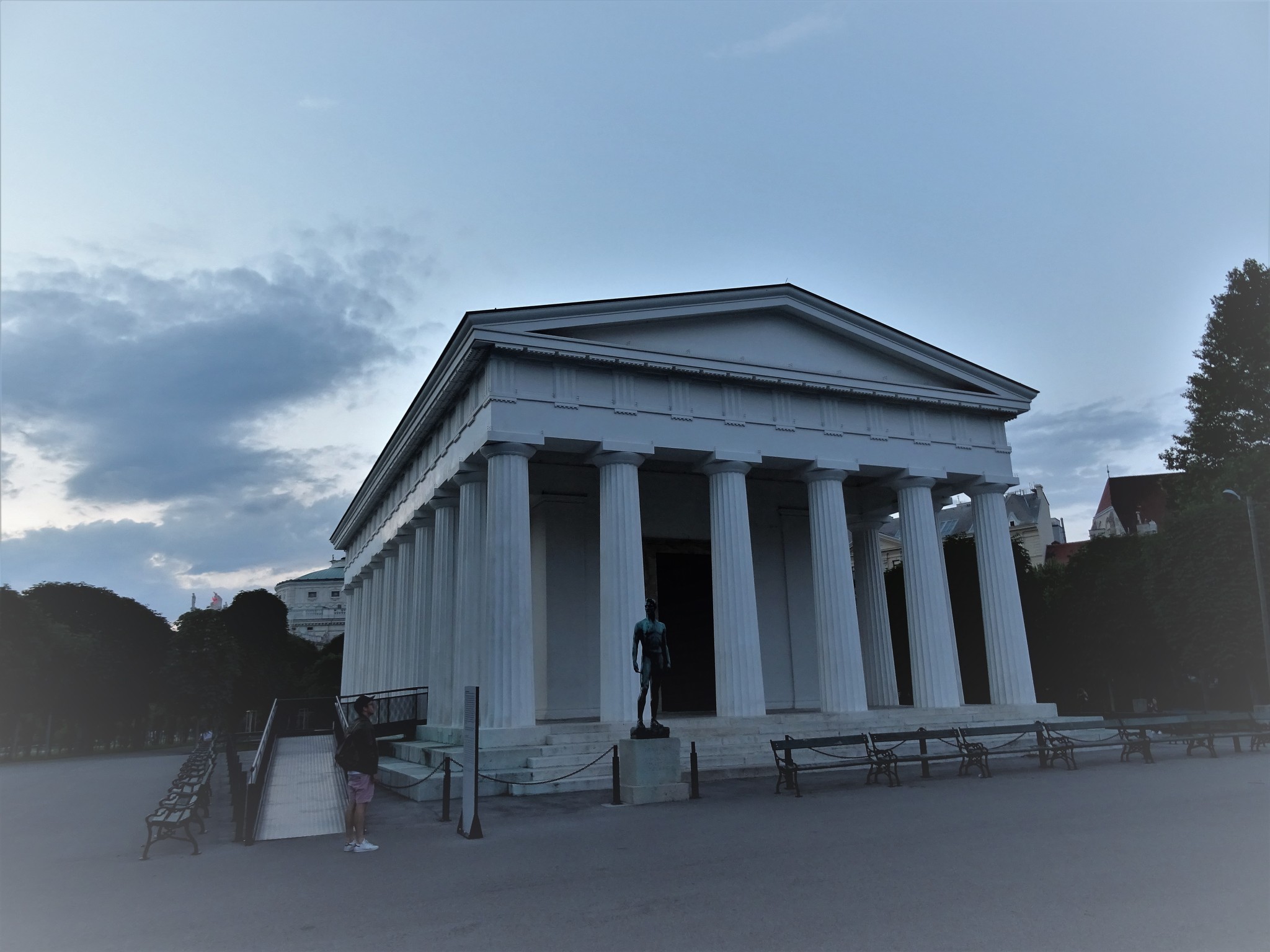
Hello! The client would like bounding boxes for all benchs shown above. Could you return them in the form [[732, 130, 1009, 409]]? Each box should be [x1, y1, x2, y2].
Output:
[[137, 730, 219, 861], [768, 715, 1270, 799]]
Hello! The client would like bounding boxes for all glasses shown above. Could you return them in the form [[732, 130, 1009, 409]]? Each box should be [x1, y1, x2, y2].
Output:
[[366, 703, 373, 706]]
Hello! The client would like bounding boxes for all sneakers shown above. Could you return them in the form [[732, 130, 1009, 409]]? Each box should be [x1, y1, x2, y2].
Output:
[[353, 838, 379, 853], [344, 840, 357, 851]]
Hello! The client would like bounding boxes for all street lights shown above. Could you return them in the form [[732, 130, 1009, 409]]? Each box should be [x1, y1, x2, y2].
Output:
[[1223, 486, 1270, 657]]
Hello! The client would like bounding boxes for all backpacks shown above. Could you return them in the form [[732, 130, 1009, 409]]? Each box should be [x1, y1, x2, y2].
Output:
[[335, 721, 366, 770]]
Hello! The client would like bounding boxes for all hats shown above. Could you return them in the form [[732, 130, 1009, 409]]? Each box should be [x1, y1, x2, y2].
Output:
[[353, 695, 375, 713]]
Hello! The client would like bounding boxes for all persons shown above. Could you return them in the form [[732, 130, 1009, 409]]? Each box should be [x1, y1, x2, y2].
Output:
[[198, 726, 215, 742], [1149, 697, 1162, 713], [335, 695, 379, 852], [1077, 688, 1091, 712], [632, 598, 671, 728]]
